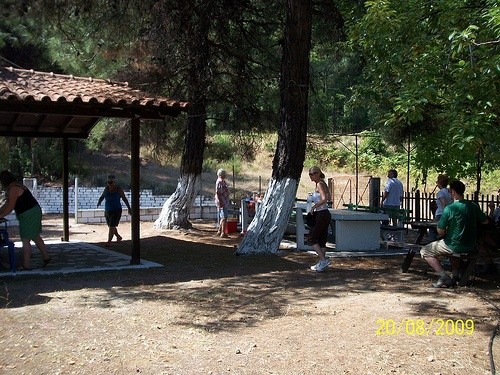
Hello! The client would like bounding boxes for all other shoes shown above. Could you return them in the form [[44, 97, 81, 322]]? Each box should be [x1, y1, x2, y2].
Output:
[[216, 233, 227, 238], [21, 266, 32, 270], [42, 257, 50, 268]]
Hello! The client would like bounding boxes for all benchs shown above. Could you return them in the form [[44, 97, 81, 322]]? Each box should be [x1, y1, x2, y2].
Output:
[[399, 242, 470, 257], [347, 204, 413, 242]]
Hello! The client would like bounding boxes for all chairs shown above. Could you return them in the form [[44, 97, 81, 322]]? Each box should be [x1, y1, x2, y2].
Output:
[[241, 200, 253, 233], [296, 207, 314, 251]]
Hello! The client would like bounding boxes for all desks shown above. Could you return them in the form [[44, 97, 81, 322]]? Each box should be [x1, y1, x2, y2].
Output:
[[330, 210, 388, 251], [402, 222, 438, 274]]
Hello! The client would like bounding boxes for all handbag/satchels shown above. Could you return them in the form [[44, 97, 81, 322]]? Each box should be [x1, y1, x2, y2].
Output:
[[306, 212, 315, 227]]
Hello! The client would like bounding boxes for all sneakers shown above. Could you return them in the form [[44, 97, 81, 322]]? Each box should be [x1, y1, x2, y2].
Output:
[[432, 273, 459, 287], [311, 260, 331, 272]]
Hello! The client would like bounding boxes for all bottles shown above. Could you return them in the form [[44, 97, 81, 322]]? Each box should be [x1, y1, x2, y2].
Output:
[[307, 193, 312, 213]]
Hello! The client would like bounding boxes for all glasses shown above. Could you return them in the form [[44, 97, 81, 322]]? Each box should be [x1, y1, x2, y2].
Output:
[[107, 181, 115, 184], [309, 172, 318, 176]]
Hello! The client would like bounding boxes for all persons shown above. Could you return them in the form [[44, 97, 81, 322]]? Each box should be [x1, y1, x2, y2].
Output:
[[0, 170, 51, 272], [216, 169, 231, 238], [419, 177, 488, 288], [309, 166, 332, 272], [429, 174, 454, 242], [97, 175, 131, 246], [381, 170, 403, 226], [239, 192, 265, 216], [488, 189, 500, 231]]
